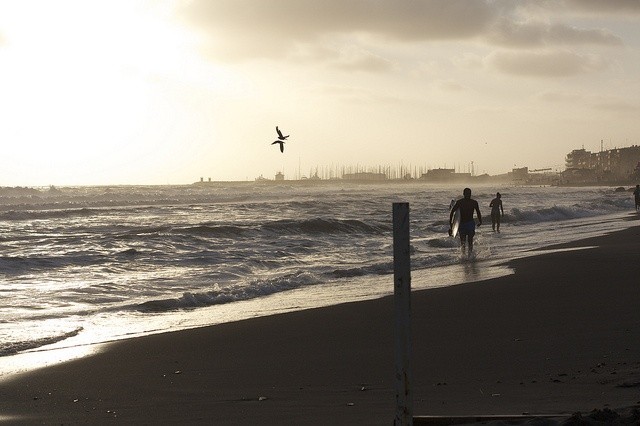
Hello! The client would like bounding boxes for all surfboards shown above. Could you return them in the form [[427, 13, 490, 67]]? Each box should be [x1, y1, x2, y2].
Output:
[[448, 199, 461, 239]]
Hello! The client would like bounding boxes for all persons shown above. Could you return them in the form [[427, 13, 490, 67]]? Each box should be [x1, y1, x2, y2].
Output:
[[489, 191, 503, 231], [448, 187, 481, 256], [632, 184, 639, 210]]
